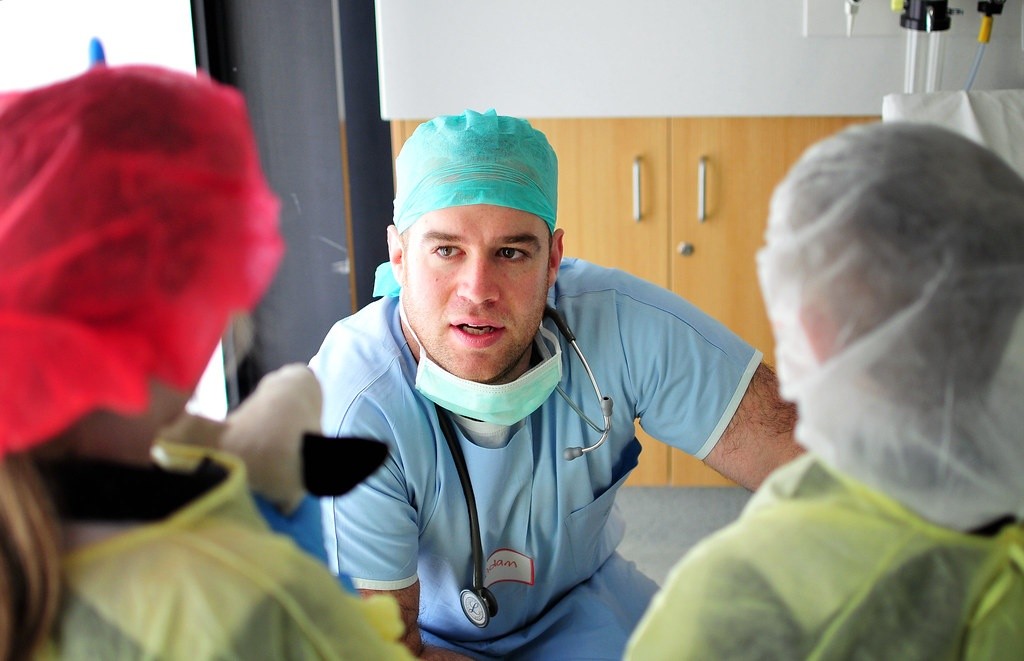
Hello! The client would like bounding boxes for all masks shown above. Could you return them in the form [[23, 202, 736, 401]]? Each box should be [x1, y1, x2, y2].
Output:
[[399, 288, 563, 426]]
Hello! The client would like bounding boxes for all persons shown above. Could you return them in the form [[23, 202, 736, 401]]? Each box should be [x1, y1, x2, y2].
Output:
[[1, 65, 421, 661], [307, 108, 808, 661], [622, 121, 1024, 661]]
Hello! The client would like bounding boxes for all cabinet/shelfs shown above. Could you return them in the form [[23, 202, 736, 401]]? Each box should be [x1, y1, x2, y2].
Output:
[[391, 115, 887, 490]]
